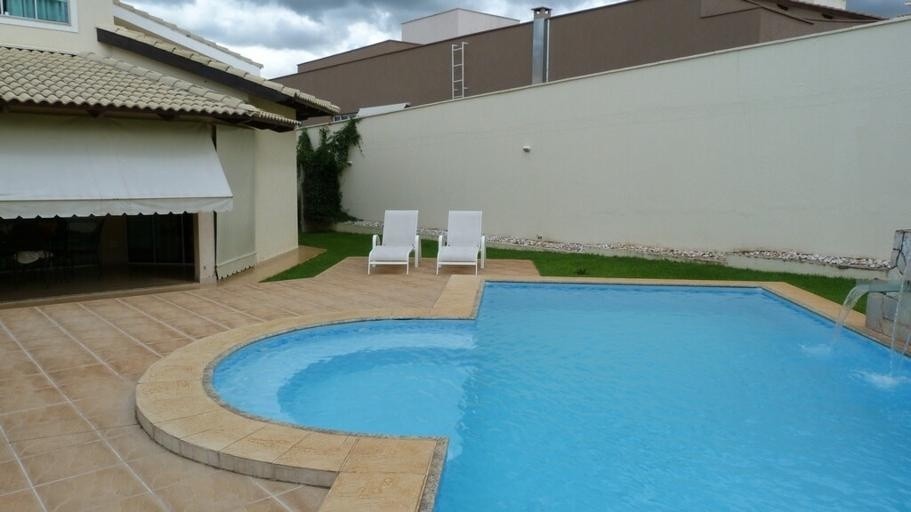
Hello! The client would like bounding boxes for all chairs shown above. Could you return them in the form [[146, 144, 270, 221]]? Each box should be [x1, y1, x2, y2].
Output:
[[367, 209, 422, 275], [436, 209, 486, 277]]
